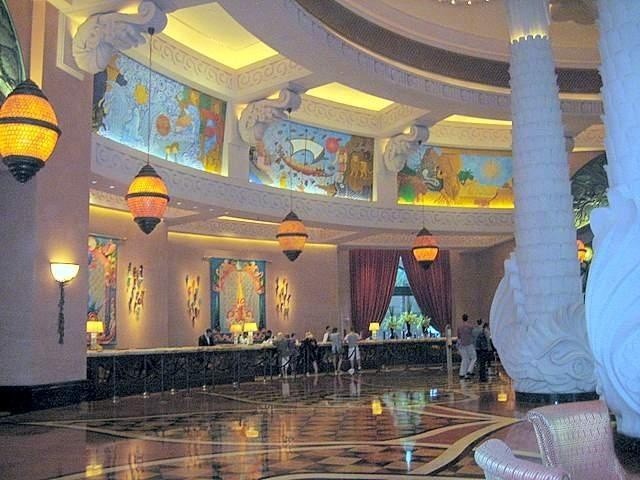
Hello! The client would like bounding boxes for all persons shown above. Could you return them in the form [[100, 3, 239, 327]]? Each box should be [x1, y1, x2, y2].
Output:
[[459, 367, 493, 413], [281, 377, 364, 412], [472, 318, 484, 367], [456, 312, 476, 380], [479, 323, 495, 367], [198, 324, 362, 376]]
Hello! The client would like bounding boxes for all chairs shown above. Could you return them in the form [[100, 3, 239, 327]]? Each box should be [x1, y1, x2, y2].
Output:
[[474, 399, 639, 480]]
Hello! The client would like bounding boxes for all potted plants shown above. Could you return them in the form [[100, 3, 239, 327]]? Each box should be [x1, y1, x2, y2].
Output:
[[386, 310, 434, 340]]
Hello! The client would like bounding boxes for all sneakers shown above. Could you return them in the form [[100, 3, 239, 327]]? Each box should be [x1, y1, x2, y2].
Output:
[[349, 369, 354, 374], [358, 367, 362, 369], [459, 375, 465, 379], [335, 372, 337, 375], [339, 372, 342, 375], [467, 373, 474, 376]]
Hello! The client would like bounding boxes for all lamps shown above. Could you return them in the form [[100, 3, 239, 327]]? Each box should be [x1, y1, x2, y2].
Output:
[[229, 323, 259, 344], [275, 106, 310, 263], [368, 322, 380, 340], [0, 0, 63, 184], [411, 140, 441, 272], [124, 26, 170, 236], [49, 260, 81, 345], [87, 319, 105, 352]]
